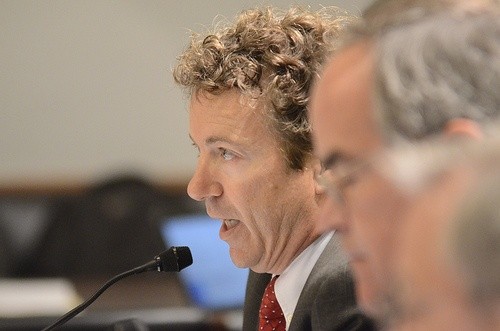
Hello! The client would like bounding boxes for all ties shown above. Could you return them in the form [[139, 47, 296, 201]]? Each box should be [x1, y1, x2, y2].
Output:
[[258, 275, 287, 331]]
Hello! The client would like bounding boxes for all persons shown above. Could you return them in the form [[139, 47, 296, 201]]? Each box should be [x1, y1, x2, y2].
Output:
[[305, 1, 500, 318], [391, 129, 499, 331], [172, 6, 380, 331]]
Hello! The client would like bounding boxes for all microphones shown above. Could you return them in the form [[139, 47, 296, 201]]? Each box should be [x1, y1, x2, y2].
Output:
[[40, 247, 193, 331]]
